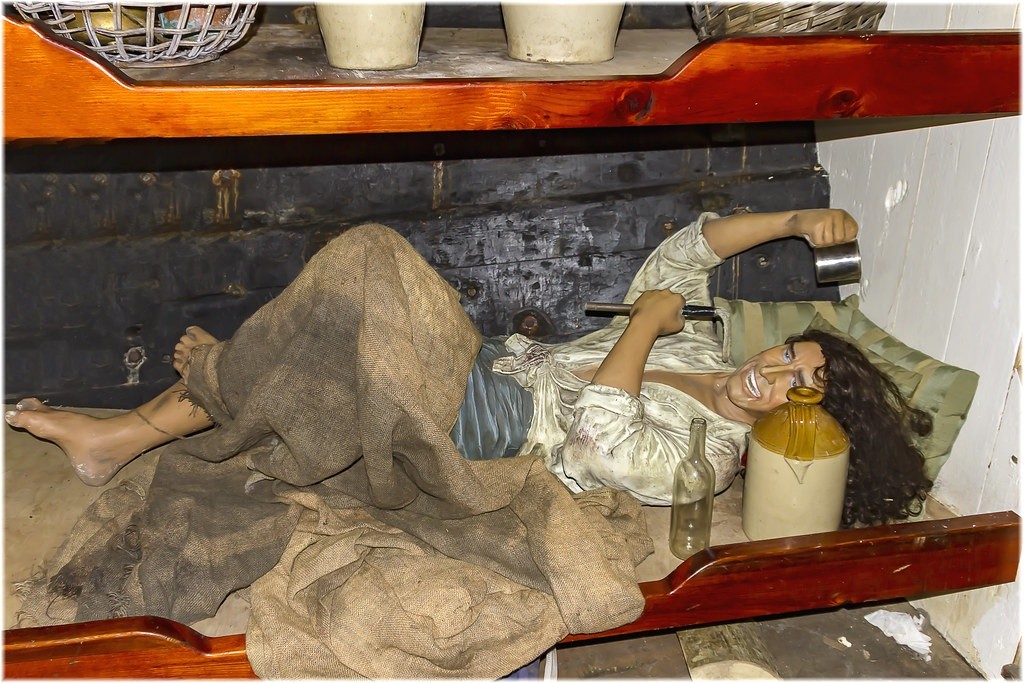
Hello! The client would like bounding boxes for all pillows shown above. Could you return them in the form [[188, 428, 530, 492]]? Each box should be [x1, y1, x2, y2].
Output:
[[713, 295, 979, 498]]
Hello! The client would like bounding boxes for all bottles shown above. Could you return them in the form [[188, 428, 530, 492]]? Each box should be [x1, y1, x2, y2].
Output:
[[670, 418, 716, 561], [741, 387, 856, 541]]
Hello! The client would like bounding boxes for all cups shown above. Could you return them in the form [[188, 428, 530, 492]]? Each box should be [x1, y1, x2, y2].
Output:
[[813, 238, 862, 283], [315, 4, 427, 70], [500, 1, 626, 64]]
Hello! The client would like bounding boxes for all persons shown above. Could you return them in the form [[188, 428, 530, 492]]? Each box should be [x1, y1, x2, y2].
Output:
[[5, 207, 933, 528]]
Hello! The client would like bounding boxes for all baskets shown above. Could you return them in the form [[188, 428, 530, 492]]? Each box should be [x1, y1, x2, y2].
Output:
[[688, 0, 888, 44], [12, 1, 261, 69]]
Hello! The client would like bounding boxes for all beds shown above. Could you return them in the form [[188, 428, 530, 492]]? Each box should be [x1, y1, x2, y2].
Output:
[[5, 302, 1019, 680]]
